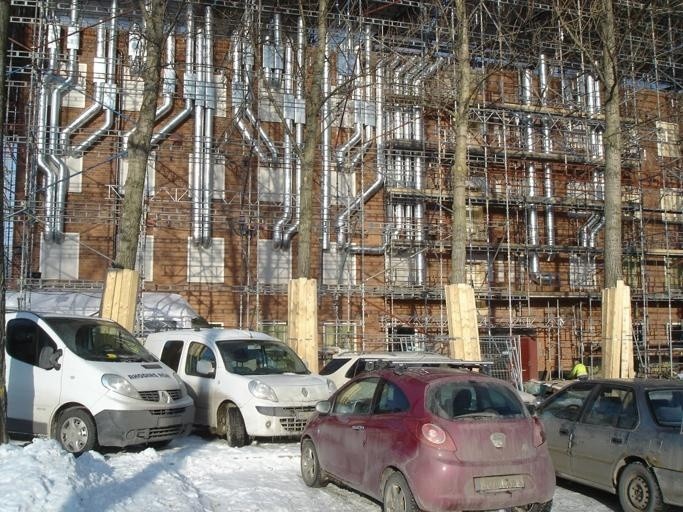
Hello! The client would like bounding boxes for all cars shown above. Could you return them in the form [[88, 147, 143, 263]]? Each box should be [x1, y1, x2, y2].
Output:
[[297, 369, 554, 511], [528, 378, 683, 512]]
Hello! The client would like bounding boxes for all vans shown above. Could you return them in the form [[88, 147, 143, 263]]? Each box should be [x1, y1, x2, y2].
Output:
[[4, 310, 194, 457], [145, 330, 336, 446]]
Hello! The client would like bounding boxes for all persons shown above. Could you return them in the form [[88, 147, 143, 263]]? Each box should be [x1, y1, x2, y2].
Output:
[[567, 360, 589, 381]]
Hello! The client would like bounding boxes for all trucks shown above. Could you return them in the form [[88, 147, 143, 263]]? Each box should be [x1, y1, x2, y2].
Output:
[[3, 293, 214, 328]]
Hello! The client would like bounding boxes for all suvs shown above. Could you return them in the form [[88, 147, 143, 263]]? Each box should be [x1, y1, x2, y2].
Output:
[[320, 354, 536, 414]]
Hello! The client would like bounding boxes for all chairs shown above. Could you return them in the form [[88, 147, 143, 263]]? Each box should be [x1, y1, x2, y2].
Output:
[[352, 398, 373, 413], [595, 396, 683, 427], [228, 349, 252, 373], [444, 388, 473, 418]]
